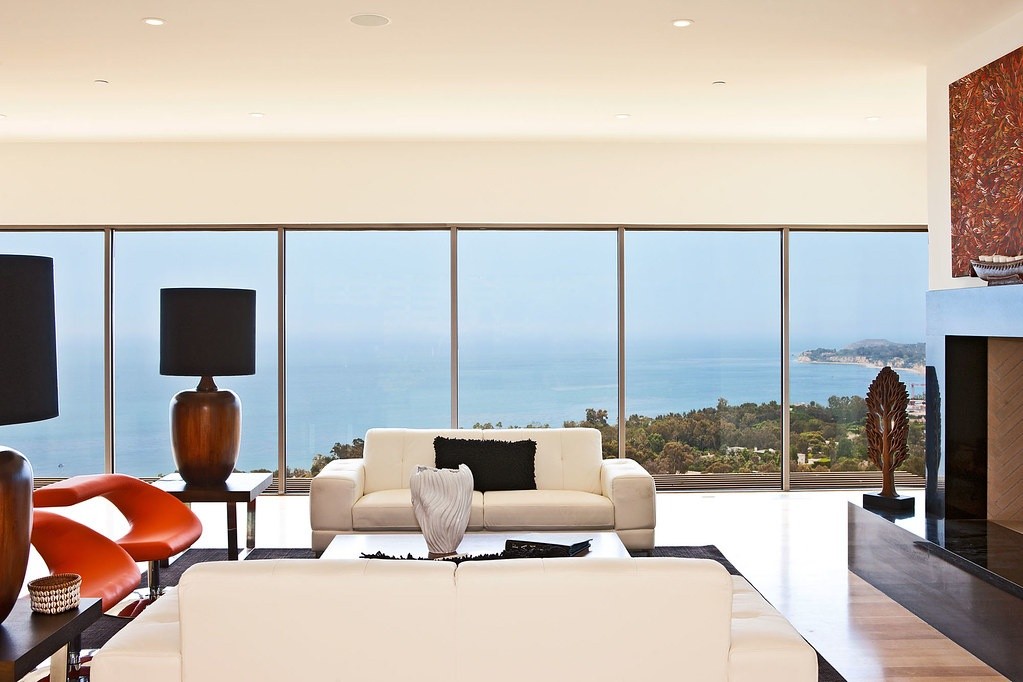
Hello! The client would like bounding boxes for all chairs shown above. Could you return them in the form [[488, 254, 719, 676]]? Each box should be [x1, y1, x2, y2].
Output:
[[33, 473, 203, 619], [21, 510, 141, 682]]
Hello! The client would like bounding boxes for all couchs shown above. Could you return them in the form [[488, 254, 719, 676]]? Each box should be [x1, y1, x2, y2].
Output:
[[310, 428, 656, 558], [90, 557, 819, 682]]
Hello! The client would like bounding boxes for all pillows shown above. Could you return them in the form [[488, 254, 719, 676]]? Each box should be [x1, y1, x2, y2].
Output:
[[434, 436, 537, 493]]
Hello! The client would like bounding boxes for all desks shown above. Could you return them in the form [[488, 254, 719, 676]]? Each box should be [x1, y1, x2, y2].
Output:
[[149, 472, 275, 569], [0, 592, 102, 682]]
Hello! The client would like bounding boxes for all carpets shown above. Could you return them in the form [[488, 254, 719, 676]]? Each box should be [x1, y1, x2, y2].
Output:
[[81, 546, 847, 682]]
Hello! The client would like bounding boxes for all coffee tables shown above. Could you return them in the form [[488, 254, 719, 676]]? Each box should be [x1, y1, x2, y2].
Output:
[[318, 530, 632, 559]]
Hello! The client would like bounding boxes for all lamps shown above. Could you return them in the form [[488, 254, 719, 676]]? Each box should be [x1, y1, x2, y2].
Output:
[[0, 253, 60, 626], [159, 288, 256, 486]]
